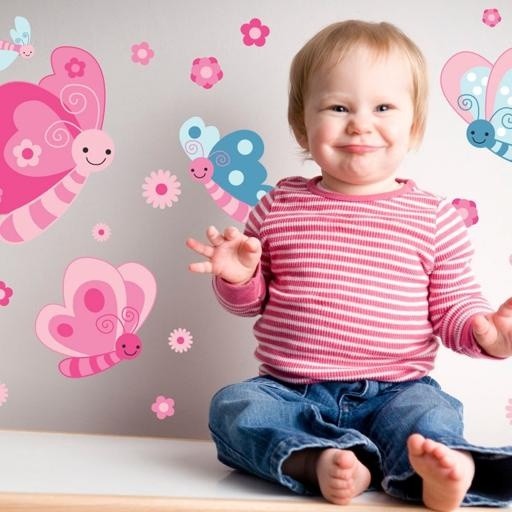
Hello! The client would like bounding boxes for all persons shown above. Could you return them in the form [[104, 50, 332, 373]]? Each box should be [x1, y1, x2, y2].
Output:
[[187, 20, 512, 511]]
